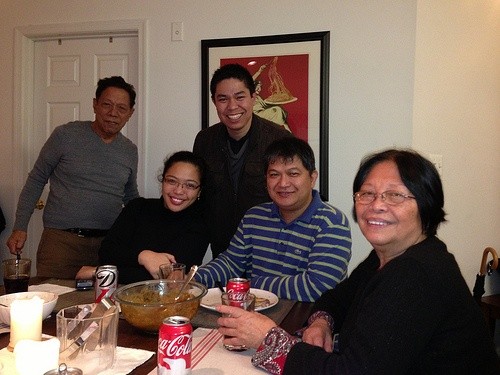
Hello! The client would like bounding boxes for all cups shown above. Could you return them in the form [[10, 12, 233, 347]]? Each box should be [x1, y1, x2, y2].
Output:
[[221, 293, 256, 352], [159, 263, 186, 282], [10, 300, 43, 351], [56, 303, 119, 374], [3, 257, 33, 293]]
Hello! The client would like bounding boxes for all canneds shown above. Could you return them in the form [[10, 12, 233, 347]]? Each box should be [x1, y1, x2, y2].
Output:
[[158, 315, 193, 375], [226, 277, 250, 309], [95, 265, 118, 304]]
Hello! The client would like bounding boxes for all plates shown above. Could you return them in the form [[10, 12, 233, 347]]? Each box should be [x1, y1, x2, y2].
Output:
[[200, 287, 279, 312]]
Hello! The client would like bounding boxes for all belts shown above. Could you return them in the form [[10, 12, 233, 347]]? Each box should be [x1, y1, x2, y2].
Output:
[[62, 228, 109, 238]]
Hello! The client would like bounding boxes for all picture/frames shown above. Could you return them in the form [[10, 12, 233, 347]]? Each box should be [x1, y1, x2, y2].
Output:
[[200, 30, 330, 203]]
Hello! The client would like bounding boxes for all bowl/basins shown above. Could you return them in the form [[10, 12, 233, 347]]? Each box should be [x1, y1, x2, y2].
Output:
[[113, 279, 207, 331], [0, 291, 59, 326]]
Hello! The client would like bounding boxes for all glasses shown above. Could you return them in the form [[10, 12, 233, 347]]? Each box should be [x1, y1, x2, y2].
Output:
[[162, 176, 201, 191], [352, 190, 416, 206]]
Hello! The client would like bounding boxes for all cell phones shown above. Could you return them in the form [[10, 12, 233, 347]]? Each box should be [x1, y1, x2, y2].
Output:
[[76, 279, 94, 290]]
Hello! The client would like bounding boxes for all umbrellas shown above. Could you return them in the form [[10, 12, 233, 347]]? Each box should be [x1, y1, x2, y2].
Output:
[[472, 247, 498, 303]]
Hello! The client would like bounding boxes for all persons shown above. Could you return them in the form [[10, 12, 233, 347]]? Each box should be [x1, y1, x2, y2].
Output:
[[165, 137, 352, 353], [6, 76, 138, 279], [192, 63, 300, 261], [103, 150, 211, 285], [216, 149, 500, 375]]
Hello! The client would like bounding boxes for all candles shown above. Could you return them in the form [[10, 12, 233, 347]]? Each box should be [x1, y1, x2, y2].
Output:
[[9, 295, 43, 348]]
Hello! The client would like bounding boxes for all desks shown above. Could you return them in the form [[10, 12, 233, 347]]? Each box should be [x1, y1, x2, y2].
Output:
[[0, 277, 314, 375]]
